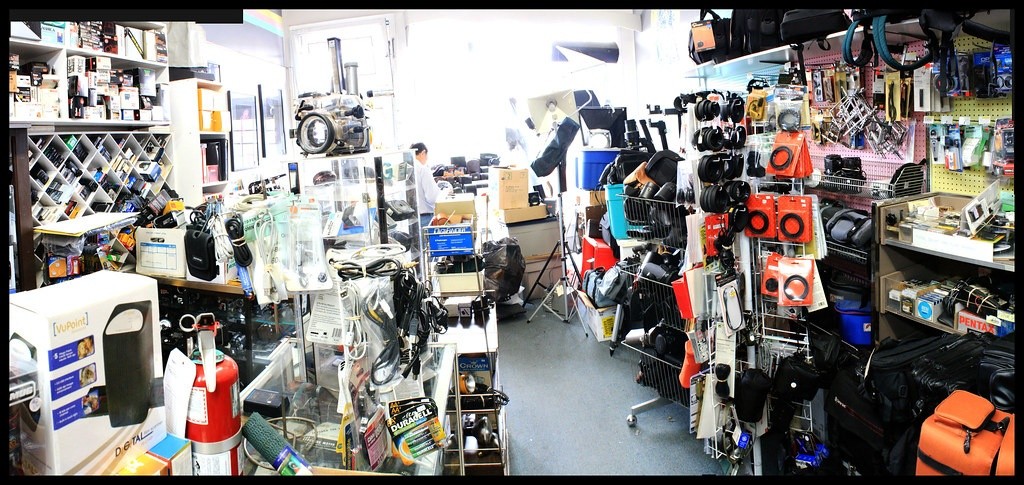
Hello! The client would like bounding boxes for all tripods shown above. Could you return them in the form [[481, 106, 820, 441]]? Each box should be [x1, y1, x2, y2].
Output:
[[522, 162, 588, 337]]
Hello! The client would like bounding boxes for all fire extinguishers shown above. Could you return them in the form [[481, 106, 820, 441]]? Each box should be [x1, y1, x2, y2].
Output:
[[180, 311, 245, 475]]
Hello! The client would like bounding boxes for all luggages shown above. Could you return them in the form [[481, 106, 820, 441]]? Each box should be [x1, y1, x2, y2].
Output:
[[915, 388, 1015, 477]]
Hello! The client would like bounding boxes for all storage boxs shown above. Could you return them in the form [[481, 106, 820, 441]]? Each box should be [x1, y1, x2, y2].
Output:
[[10, 270, 193, 476], [957, 308, 1014, 338], [581, 235, 619, 280], [916, 281, 959, 324], [900, 276, 956, 316], [498, 203, 567, 300], [603, 184, 645, 239], [577, 148, 623, 190], [198, 88, 231, 133], [885, 267, 930, 311], [439, 268, 486, 293], [186, 256, 238, 285], [135, 226, 186, 278], [575, 290, 617, 342], [10, 21, 186, 283], [434, 193, 477, 238], [427, 214, 474, 251], [206, 165, 218, 183], [487, 165, 529, 209]]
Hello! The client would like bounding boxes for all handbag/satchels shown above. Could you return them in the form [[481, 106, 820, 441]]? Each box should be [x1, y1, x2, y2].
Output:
[[819, 329, 1015, 469], [684, 7, 732, 67], [582, 254, 640, 309]]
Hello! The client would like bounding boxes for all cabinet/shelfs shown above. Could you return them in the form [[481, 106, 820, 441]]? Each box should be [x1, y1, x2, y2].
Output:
[[287, 149, 427, 420], [11, 22, 171, 126], [10, 131, 176, 294], [771, 165, 1015, 352], [422, 223, 510, 476], [150, 78, 230, 223]]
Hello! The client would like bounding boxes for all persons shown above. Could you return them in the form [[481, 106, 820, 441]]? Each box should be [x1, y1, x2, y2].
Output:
[[410, 143, 454, 274]]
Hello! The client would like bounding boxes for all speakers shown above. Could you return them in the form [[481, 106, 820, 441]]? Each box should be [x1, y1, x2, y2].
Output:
[[528, 191, 540, 206]]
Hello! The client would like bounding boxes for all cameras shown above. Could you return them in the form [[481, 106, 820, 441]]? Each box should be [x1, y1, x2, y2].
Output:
[[288, 90, 371, 158]]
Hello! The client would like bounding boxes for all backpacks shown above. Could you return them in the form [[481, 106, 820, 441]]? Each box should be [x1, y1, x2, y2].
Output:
[[780, 9, 853, 86], [729, 3, 783, 53], [842, 2, 1005, 98]]
